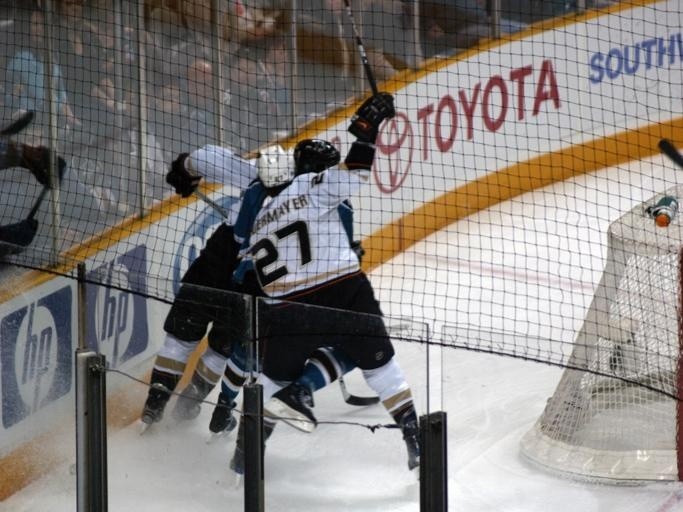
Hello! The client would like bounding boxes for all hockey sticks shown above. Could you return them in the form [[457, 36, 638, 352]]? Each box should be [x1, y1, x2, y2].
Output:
[[338, 377, 380, 406]]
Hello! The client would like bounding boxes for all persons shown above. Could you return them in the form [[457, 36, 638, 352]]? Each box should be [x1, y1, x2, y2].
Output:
[[229, 91, 421, 476], [139, 143, 257, 437], [0, 2, 605, 248], [207, 146, 363, 432]]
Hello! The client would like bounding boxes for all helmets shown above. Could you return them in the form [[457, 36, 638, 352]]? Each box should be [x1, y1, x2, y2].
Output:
[[294, 139, 341, 177], [258, 146, 289, 188]]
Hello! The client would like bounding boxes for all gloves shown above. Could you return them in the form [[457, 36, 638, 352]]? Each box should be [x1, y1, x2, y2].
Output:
[[348, 91, 395, 144], [165, 153, 202, 198], [30, 144, 68, 191]]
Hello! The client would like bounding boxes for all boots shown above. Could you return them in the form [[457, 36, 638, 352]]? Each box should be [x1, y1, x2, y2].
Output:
[[229, 415, 276, 474], [172, 371, 217, 422], [210, 394, 236, 432], [141, 363, 183, 425], [272, 379, 318, 428], [387, 401, 422, 469]]
